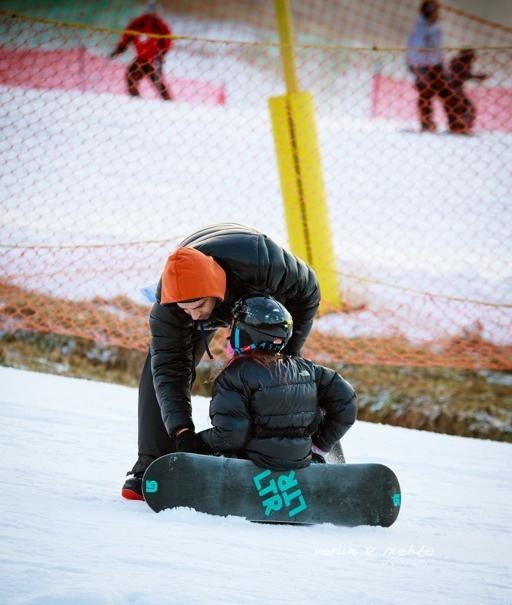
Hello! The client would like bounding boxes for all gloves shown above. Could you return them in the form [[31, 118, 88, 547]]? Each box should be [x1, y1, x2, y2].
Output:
[[175, 429, 213, 455]]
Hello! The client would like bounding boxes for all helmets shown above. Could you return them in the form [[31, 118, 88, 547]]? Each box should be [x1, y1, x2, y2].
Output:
[[228, 290, 295, 357]]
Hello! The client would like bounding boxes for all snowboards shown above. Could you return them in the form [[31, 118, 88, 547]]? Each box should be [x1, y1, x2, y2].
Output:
[[142, 452, 401, 526]]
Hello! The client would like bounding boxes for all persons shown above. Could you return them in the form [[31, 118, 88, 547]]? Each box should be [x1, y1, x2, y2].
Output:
[[196, 294, 358, 475], [448, 45, 494, 136], [404, 1, 465, 135], [121, 220, 322, 500], [106, 1, 175, 100]]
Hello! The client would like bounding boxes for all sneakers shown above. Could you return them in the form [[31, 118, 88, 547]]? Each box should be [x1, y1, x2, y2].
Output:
[[121, 476, 144, 501]]
